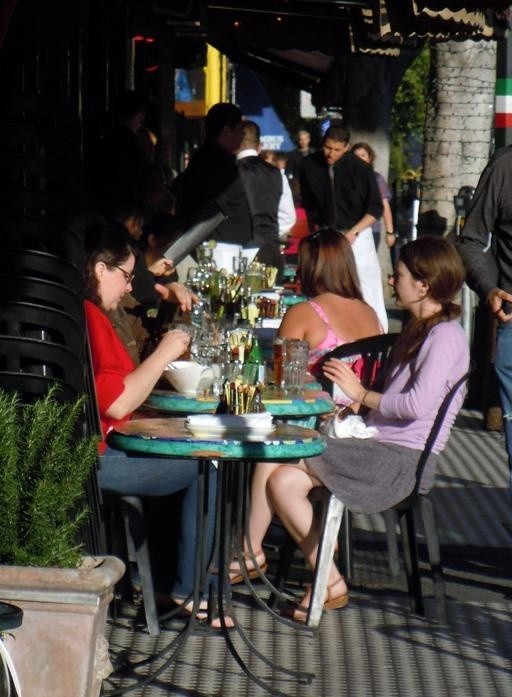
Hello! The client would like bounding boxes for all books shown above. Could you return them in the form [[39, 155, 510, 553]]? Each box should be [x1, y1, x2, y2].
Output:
[[163, 211, 225, 268]]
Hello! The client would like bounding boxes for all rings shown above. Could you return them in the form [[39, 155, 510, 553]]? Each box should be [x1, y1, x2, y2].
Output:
[[184, 290, 192, 298]]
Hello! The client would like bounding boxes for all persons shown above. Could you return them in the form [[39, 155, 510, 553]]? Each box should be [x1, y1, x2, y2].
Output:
[[349, 142, 395, 253], [153, 283, 199, 313], [284, 179, 312, 265], [261, 150, 275, 163], [285, 130, 316, 179], [172, 103, 253, 243], [299, 124, 388, 335], [236, 121, 296, 275], [114, 209, 174, 299], [454, 145, 512, 472], [84, 240, 236, 630], [278, 230, 385, 406], [229, 236, 470, 618], [141, 210, 177, 268], [275, 151, 289, 169]]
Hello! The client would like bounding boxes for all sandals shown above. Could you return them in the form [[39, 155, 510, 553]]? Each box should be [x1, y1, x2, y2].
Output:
[[285, 575, 350, 620], [208, 549, 269, 586], [168, 599, 234, 633]]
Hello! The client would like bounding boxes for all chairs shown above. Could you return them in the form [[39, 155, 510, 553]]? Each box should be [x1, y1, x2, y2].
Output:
[[0, 248, 158, 635], [252, 334, 434, 582], [307, 357, 475, 626]]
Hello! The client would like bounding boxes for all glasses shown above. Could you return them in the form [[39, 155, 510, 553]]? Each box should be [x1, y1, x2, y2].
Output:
[[98, 259, 137, 286]]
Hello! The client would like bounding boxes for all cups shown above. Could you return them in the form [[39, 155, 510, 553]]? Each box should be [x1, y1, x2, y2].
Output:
[[191, 300, 209, 331], [186, 267, 203, 294], [285, 339, 309, 397]]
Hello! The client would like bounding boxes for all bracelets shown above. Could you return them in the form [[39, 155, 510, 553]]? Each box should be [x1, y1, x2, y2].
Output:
[[385, 229, 393, 237], [360, 388, 372, 407], [386, 231, 394, 235], [384, 229, 397, 238]]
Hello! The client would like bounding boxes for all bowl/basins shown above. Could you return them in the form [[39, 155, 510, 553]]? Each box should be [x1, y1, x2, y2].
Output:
[[162, 361, 219, 395]]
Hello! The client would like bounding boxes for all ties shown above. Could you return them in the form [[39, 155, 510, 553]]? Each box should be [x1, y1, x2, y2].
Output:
[[326, 163, 339, 225]]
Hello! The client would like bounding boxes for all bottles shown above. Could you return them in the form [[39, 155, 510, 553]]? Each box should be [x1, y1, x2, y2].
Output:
[[257, 317, 263, 329], [237, 287, 249, 325], [248, 335, 266, 387], [217, 277, 229, 324], [246, 287, 251, 305]]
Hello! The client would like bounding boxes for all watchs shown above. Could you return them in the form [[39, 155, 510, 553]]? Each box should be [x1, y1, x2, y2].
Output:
[[350, 229, 360, 237]]
